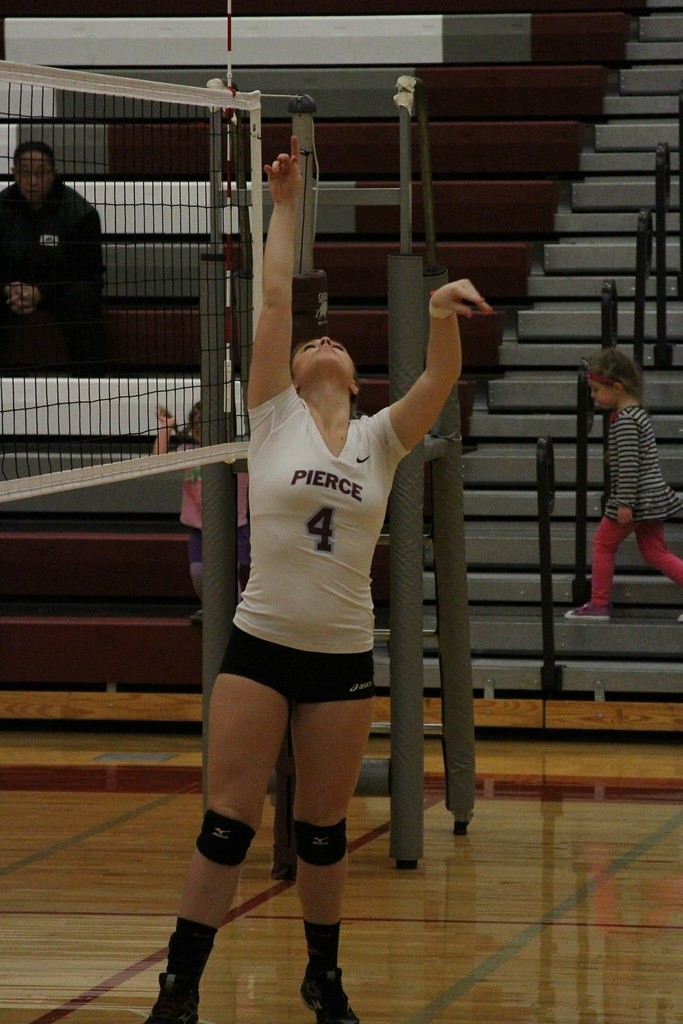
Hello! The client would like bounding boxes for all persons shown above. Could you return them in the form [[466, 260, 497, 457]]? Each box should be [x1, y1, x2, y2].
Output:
[[562, 344, 683, 627], [1, 141, 105, 376], [142, 131, 499, 1024], [152, 401, 249, 620]]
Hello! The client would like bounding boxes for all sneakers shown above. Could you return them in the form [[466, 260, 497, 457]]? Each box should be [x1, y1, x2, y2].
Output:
[[139, 976, 201, 1023], [565, 602, 612, 624], [296, 968, 363, 1024]]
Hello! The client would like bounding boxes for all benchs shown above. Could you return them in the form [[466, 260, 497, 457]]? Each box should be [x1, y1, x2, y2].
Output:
[[0, 0, 683, 797]]
